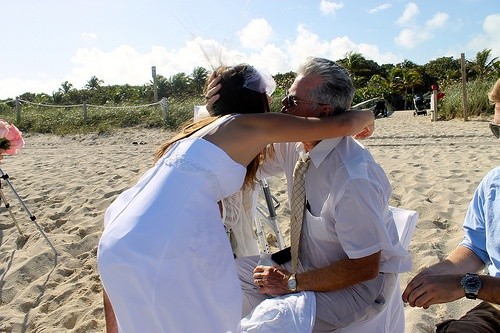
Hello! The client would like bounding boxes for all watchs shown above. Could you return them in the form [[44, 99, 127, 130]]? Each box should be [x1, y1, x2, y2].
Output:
[[460, 273, 482, 300], [288, 273, 298, 294]]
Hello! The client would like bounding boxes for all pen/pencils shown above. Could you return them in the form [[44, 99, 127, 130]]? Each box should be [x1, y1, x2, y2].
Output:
[[304, 199, 312, 212]]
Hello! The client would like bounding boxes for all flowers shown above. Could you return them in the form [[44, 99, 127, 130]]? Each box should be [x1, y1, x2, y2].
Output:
[[0, 118, 25, 159]]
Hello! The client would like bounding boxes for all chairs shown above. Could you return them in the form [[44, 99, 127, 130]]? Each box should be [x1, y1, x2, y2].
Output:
[[330, 206, 420, 333]]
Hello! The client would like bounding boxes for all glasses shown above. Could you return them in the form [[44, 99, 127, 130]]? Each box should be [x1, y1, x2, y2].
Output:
[[488, 121, 500, 139], [285, 89, 329, 108]]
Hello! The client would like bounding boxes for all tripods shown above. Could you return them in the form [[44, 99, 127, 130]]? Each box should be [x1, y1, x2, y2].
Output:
[[0, 169, 59, 255]]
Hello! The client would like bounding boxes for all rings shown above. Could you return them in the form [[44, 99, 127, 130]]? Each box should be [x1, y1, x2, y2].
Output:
[[205, 95, 210, 100], [257, 279, 259, 285]]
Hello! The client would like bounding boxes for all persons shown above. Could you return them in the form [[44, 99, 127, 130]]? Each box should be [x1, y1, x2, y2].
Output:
[[203, 57, 412, 333], [97, 63, 375, 333], [401, 79, 500, 333]]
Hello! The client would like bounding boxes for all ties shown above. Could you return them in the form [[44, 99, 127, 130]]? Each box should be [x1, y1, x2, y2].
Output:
[[289, 156, 312, 273]]
